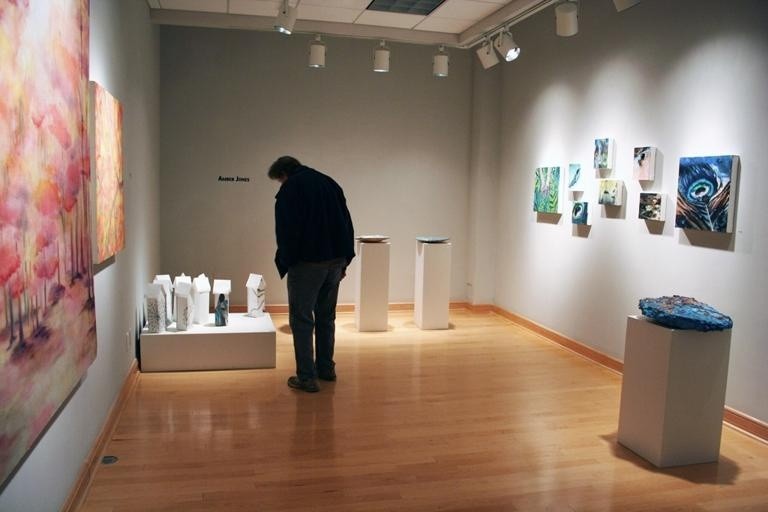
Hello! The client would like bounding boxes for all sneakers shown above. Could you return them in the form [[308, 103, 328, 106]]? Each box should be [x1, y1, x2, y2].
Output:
[[316, 373, 337, 382], [288, 376, 320, 392]]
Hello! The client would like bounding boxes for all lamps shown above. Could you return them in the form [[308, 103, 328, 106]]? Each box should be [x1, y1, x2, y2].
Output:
[[272, 0, 643, 80]]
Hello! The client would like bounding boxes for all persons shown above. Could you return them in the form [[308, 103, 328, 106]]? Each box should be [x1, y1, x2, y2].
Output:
[[639, 193, 662, 220], [637, 153, 648, 180], [214, 294, 227, 327], [266, 156, 355, 394]]
